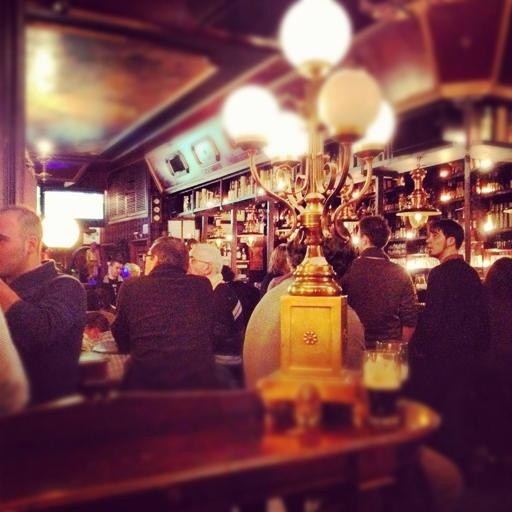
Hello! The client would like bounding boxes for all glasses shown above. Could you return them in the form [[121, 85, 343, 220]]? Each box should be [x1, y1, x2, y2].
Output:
[[189, 257, 204, 266], [145, 253, 154, 258]]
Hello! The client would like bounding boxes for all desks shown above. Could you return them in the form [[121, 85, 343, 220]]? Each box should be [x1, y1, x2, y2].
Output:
[[0, 353, 464, 512]]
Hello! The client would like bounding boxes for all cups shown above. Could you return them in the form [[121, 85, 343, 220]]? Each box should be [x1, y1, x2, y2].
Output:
[[374, 337, 411, 406], [358, 348, 403, 425]]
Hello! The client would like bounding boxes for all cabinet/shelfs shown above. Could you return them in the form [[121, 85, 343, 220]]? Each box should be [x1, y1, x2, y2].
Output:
[[160, 81, 512, 275]]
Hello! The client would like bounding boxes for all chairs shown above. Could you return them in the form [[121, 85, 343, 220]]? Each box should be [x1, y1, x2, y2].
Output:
[[85, 284, 115, 311]]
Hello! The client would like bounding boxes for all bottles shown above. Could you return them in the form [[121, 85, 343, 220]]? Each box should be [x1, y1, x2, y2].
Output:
[[384, 171, 512, 291], [208, 203, 268, 260]]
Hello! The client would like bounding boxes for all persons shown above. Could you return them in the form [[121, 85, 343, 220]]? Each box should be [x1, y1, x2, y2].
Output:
[[0, 205, 89, 405], [474, 257, 511, 483], [337, 215, 420, 355], [407, 219, 484, 464]]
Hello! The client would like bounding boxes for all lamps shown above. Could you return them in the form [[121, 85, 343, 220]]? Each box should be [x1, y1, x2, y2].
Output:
[[221, 0, 397, 447], [35, 139, 53, 184]]
